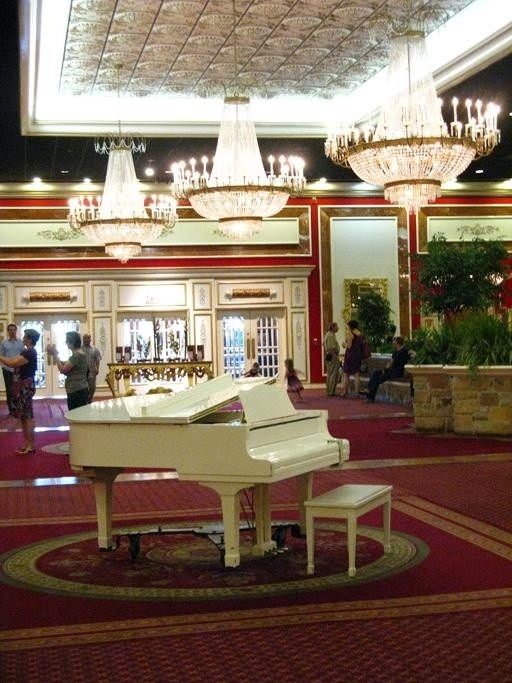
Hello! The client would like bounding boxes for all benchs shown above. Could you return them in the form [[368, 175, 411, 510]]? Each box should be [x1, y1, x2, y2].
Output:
[[347, 368, 413, 408]]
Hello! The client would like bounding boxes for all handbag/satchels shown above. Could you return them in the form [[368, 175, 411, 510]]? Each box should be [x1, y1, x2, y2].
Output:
[[362, 341, 370, 357]]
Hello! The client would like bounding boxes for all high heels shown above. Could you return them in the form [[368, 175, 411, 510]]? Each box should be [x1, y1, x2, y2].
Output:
[[14, 448, 36, 456]]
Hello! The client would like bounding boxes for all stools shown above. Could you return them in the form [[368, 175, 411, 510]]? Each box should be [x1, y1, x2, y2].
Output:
[[301, 482, 396, 583]]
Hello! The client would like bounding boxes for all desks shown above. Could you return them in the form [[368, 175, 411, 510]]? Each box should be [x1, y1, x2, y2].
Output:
[[103, 359, 215, 396]]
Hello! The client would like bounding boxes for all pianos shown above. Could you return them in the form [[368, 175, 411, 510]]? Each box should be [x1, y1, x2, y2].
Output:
[[64, 371, 349, 569]]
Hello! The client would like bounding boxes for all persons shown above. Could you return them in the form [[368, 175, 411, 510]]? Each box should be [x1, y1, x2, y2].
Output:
[[1, 327, 41, 454], [82, 331, 104, 403], [283, 357, 304, 402], [339, 319, 363, 397], [1, 323, 26, 416], [322, 321, 342, 395], [47, 330, 90, 410], [358, 336, 409, 403]]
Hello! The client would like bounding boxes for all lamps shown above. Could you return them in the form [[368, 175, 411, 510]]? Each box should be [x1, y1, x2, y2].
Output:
[[170, 1, 313, 239], [63, 48, 181, 268], [320, 0, 505, 215]]
